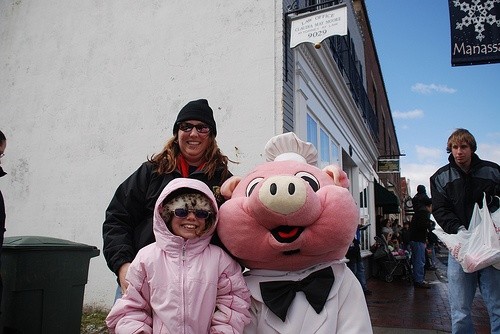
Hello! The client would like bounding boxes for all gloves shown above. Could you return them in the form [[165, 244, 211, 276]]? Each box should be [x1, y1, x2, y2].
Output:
[[472, 185, 492, 205]]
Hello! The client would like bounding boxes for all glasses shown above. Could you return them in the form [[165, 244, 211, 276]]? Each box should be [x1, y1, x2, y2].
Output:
[[0, 153, 5, 158], [169, 207, 212, 220], [177, 122, 212, 133]]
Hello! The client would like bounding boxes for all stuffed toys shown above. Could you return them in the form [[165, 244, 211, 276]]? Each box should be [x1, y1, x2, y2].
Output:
[[216, 131, 375, 334]]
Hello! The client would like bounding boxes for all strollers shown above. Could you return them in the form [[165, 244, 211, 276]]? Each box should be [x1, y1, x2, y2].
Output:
[[374, 233, 413, 284]]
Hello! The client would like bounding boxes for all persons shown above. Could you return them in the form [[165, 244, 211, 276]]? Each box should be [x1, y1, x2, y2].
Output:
[[102, 98, 244, 304], [429, 128, 500, 334], [374, 184, 436, 290], [344, 222, 373, 295], [0, 128, 7, 246], [104, 177, 253, 334]]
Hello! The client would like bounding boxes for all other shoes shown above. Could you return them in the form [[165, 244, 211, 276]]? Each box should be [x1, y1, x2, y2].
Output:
[[414, 283, 431, 289]]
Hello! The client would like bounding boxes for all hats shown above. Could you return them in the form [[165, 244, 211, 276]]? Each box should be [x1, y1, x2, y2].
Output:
[[173, 99, 217, 136], [160, 193, 214, 231]]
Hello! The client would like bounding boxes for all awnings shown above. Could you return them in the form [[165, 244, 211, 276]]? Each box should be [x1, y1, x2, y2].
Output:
[[373, 179, 401, 215]]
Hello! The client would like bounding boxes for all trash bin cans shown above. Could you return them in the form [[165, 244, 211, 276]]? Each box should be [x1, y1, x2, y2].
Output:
[[0, 235, 100, 334]]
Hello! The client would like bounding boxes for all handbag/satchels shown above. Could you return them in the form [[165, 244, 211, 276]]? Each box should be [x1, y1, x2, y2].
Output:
[[345, 238, 360, 259]]
